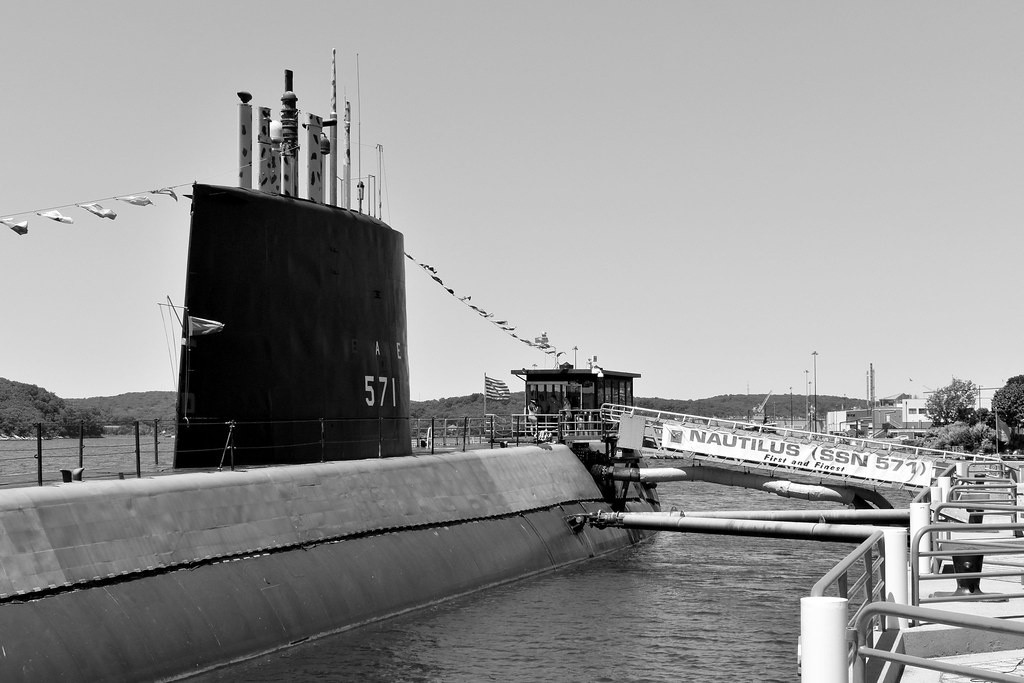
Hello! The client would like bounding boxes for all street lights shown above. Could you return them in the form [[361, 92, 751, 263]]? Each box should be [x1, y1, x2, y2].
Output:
[[789, 386, 795, 429], [811, 351, 818, 432], [804, 368, 810, 431]]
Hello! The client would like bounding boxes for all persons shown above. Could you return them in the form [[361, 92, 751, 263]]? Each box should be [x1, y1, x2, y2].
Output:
[[562, 397, 572, 437], [549, 395, 562, 421], [538, 394, 548, 414], [528, 399, 538, 435]]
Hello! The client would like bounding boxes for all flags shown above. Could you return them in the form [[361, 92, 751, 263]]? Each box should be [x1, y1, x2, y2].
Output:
[[79, 203, 117, 221], [189, 316, 225, 336], [38, 210, 74, 224], [997, 417, 1011, 445], [0, 218, 29, 236], [153, 188, 177, 201], [118, 195, 153, 206], [408, 254, 566, 357], [485, 376, 511, 405]]
